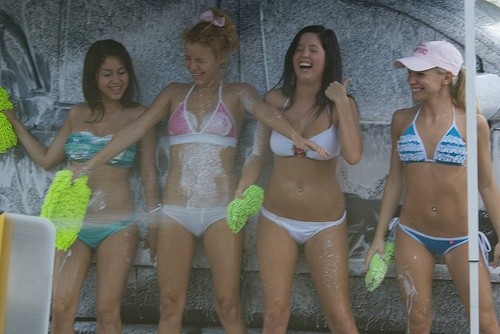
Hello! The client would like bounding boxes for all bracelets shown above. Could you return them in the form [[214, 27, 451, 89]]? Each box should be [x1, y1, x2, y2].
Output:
[[82, 163, 89, 174]]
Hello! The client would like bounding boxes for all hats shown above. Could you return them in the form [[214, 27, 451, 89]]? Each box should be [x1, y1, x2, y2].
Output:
[[394, 40, 464, 75]]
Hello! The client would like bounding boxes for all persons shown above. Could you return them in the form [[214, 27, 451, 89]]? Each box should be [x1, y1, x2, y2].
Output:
[[233, 26, 362, 334], [366, 40, 500, 334], [63, 8, 333, 334], [0, 39, 162, 334]]
